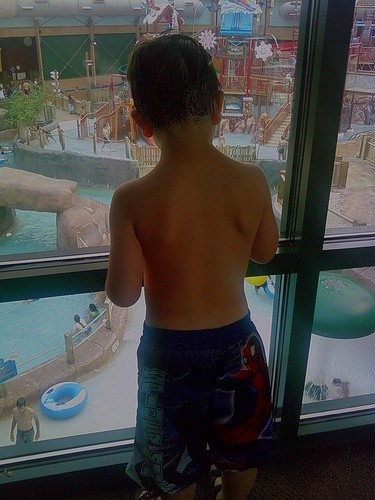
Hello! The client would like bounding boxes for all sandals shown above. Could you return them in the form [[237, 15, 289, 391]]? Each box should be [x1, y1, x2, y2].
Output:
[[136, 487, 161, 497], [209, 466, 224, 500]]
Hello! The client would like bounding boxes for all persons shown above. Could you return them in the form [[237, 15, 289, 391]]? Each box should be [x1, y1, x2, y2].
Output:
[[0, 77, 38, 100], [83, 303, 99, 319], [102, 123, 115, 152], [30, 126, 56, 148], [10, 397, 39, 445], [254, 275, 273, 296], [57, 125, 65, 150], [105, 34, 279, 500], [68, 94, 75, 114], [80, 106, 86, 120], [281, 70, 292, 88], [276, 136, 288, 160], [72, 314, 88, 345], [218, 133, 225, 146], [332, 378, 350, 399]]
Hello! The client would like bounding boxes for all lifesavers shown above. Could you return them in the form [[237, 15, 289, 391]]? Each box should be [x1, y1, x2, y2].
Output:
[[41, 381, 88, 419], [264, 281, 275, 298]]
